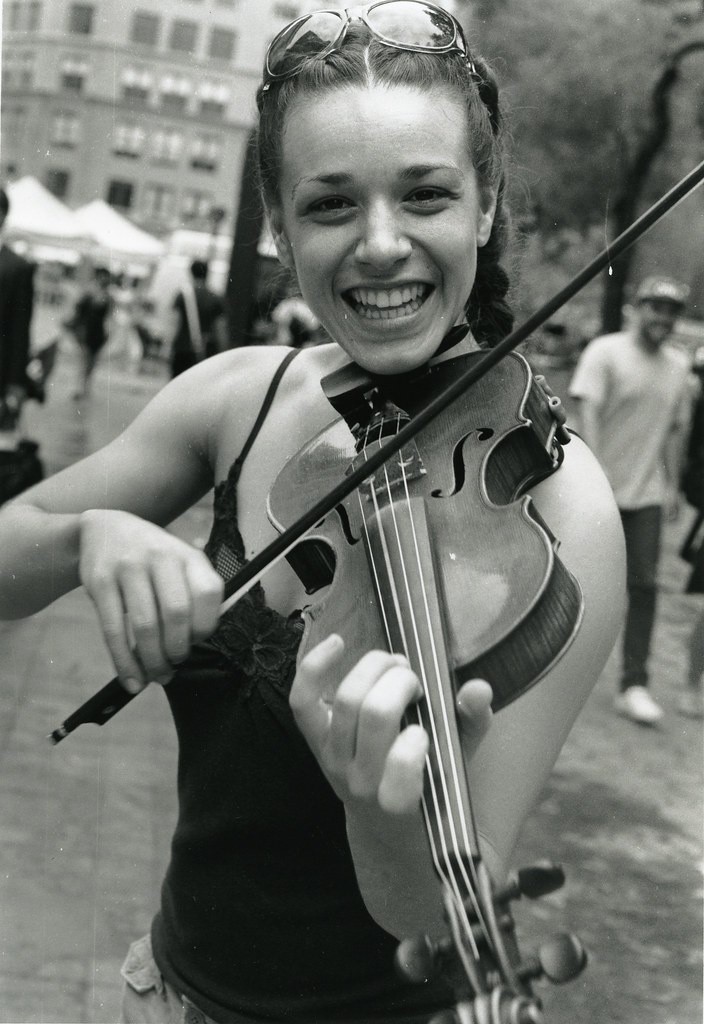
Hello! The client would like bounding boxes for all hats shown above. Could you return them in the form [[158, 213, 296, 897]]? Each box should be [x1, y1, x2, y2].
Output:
[[635, 277, 687, 312]]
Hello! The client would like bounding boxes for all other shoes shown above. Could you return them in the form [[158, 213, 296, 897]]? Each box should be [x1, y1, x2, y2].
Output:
[[614, 686, 663, 722]]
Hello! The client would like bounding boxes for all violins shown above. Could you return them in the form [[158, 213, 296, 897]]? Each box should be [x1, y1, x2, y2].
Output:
[[264, 323, 588, 1024]]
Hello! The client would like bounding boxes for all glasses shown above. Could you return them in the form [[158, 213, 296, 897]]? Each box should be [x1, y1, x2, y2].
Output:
[[260, 0, 477, 96]]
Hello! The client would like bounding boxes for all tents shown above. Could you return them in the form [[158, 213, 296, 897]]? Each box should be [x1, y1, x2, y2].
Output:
[[1, 175, 164, 297]]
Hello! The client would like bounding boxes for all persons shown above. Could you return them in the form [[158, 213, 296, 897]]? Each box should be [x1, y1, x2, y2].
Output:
[[62, 267, 115, 403], [272, 281, 321, 351], [0, 187, 33, 495], [568, 274, 704, 723], [168, 261, 232, 384], [0, 0, 629, 1024]]
